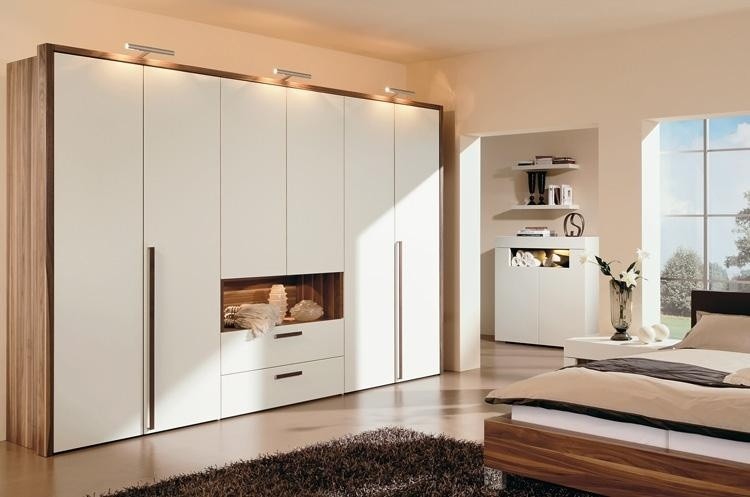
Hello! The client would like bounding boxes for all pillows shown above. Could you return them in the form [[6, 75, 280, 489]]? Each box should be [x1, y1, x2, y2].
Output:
[[672, 313, 750, 354]]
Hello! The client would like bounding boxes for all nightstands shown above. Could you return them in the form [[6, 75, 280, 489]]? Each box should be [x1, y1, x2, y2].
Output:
[[564, 335, 682, 367]]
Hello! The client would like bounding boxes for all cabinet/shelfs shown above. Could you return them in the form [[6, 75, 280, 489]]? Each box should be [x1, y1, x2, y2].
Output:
[[510, 164, 579, 210], [494, 236, 599, 348], [344, 94, 440, 398], [219, 76, 345, 284], [220, 314, 344, 421], [6, 43, 220, 459]]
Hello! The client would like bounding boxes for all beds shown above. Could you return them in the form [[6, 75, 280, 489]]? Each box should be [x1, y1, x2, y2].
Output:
[[484, 290, 750, 497]]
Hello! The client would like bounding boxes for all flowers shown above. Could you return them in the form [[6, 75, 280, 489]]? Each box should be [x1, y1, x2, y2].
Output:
[[594, 246, 651, 319]]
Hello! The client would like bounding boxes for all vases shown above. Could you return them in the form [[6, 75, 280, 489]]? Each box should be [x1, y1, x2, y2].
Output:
[[609, 279, 633, 341]]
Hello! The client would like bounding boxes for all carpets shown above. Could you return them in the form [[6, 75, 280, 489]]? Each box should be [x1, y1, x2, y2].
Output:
[[85, 427, 610, 497]]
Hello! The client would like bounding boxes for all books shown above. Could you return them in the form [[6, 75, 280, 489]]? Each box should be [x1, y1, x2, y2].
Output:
[[518, 155, 576, 207]]
